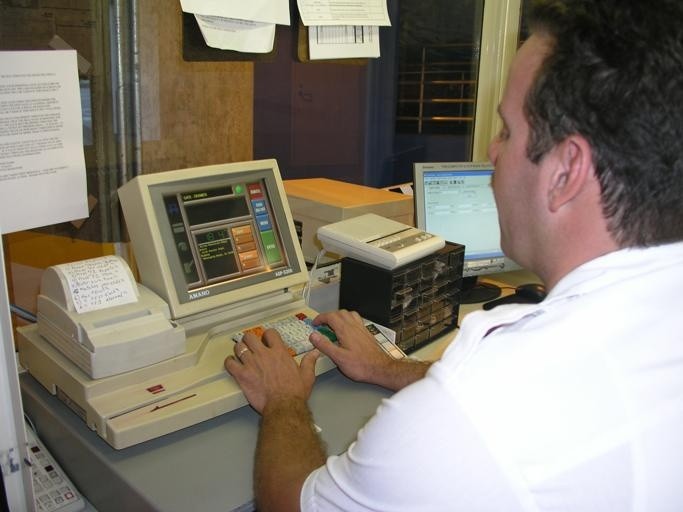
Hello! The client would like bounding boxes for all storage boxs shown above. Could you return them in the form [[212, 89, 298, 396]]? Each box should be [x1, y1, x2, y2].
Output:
[[282, 177, 414, 267]]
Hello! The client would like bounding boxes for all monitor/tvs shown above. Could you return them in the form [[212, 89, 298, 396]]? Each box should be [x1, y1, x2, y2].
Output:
[[413, 161, 525, 305], [115, 158, 310, 322]]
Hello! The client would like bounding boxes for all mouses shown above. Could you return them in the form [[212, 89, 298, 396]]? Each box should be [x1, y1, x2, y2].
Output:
[[516, 283, 548, 300]]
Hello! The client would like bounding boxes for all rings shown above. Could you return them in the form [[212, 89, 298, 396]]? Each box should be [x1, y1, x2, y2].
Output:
[[238, 347, 248, 359]]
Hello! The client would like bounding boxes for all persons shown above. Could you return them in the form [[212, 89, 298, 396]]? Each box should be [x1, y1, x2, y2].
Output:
[[221, 0, 682, 511]]
